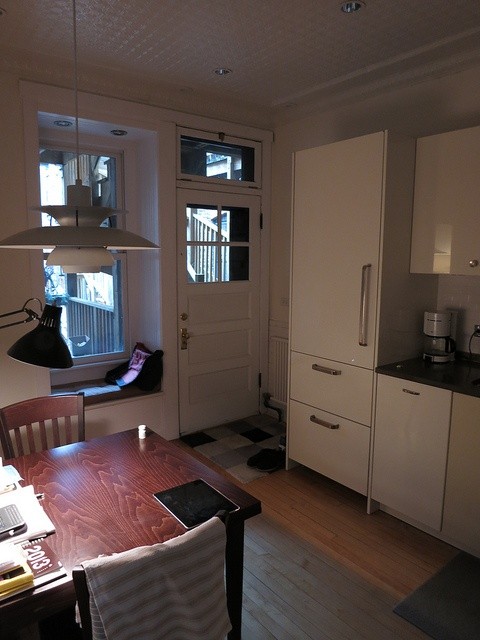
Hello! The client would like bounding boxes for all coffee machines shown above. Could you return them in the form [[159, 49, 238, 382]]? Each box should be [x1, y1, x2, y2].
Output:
[[422, 309, 458, 365]]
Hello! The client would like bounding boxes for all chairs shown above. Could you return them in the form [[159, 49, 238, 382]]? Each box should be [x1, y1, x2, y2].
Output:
[[0, 392, 86, 461], [37, 509, 232, 640]]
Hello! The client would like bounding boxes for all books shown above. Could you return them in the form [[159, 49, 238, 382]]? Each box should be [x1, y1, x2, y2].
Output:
[[0, 457, 68, 601]]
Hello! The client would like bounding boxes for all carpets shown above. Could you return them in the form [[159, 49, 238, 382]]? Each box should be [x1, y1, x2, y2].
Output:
[[180, 414, 287, 485]]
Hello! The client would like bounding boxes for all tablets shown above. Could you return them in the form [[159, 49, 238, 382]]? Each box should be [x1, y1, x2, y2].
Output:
[[150, 477, 241, 534]]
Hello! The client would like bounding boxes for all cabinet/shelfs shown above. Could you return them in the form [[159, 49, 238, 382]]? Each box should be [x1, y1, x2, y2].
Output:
[[409, 125, 480, 276], [370, 352, 480, 558], [285, 130, 442, 515]]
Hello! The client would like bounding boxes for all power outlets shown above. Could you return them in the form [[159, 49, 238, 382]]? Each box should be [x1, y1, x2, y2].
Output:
[[473, 325, 480, 337]]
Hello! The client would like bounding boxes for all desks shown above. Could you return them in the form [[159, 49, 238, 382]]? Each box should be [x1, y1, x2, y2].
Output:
[[0, 426, 263, 640]]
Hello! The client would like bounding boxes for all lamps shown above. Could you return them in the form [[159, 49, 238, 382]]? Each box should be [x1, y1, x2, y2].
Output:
[[0, 297, 74, 370], [1, 0, 164, 275]]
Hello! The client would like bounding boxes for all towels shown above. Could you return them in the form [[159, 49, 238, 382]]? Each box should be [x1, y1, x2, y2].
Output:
[[78, 515, 233, 640]]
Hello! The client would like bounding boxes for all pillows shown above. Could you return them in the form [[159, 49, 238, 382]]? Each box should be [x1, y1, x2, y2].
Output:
[[105, 342, 164, 390]]
